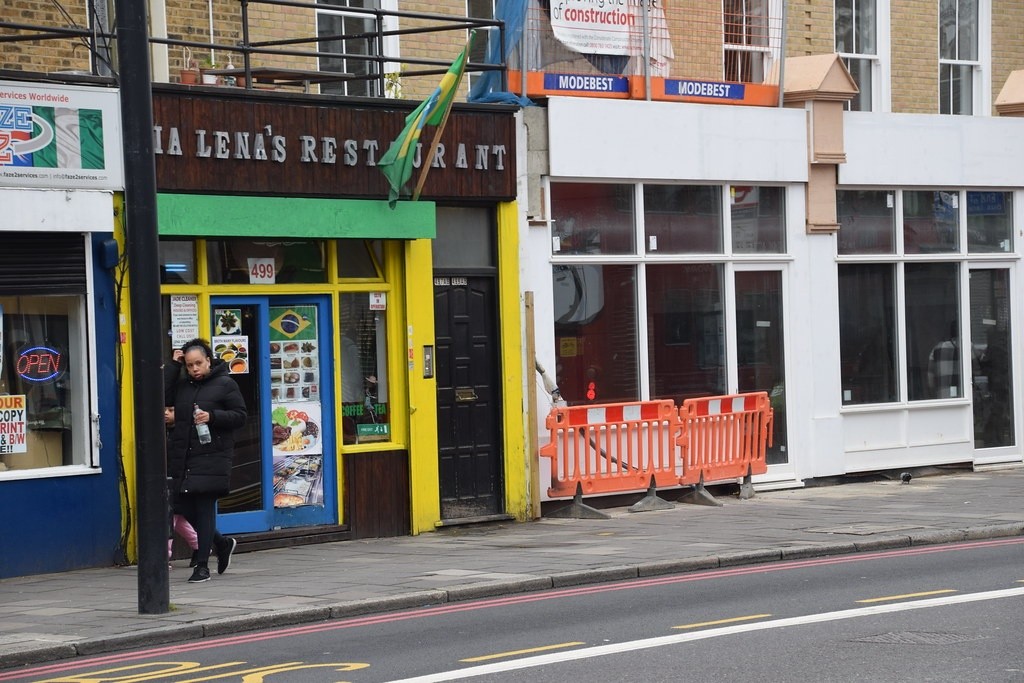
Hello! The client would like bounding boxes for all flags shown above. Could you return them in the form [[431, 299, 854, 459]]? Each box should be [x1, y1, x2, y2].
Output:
[[376, 28, 477, 210]]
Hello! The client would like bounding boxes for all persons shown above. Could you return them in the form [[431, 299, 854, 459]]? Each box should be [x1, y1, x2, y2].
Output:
[[165, 338, 248, 583], [927, 323, 989, 435]]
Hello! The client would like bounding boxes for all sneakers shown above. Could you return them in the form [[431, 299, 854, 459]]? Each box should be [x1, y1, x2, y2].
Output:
[[188, 565, 211, 583], [190, 547, 213, 567], [214, 538, 236, 574]]
